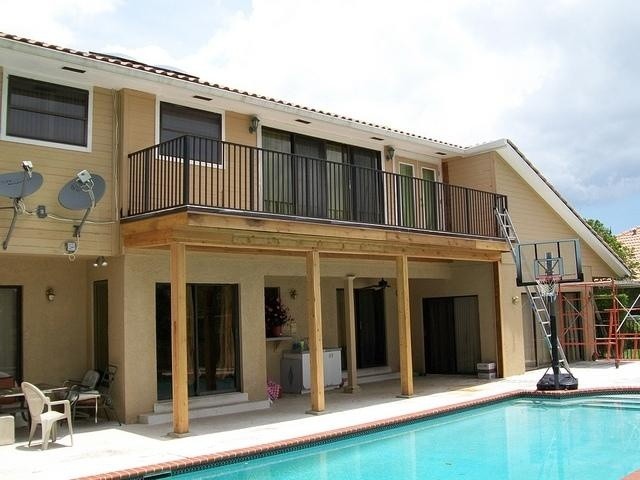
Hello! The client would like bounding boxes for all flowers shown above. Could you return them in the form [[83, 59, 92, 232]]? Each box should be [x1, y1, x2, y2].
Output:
[[265, 296, 291, 327]]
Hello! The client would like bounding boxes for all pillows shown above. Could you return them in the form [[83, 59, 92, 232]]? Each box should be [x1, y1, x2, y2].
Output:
[[0, 375, 17, 405]]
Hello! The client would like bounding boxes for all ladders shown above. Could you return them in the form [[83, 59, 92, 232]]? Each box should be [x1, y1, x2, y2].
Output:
[[494, 206, 571, 375]]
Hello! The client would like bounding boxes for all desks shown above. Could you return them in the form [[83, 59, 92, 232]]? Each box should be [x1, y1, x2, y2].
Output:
[[0, 382, 68, 438]]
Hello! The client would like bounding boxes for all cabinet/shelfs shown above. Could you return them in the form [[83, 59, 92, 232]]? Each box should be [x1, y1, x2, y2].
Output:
[[283, 348, 343, 394]]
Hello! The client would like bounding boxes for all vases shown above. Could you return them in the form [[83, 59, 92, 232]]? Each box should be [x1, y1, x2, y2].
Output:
[[270, 325, 281, 337]]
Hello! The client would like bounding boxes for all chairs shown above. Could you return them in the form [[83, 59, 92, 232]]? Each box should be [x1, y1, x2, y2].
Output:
[[57, 364, 122, 426], [0, 370, 28, 423], [21, 381, 74, 450]]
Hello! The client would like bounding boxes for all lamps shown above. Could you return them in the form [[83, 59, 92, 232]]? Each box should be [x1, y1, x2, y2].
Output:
[[387, 147, 395, 160], [249, 117, 260, 132], [93, 256, 108, 267], [46, 288, 56, 301]]
